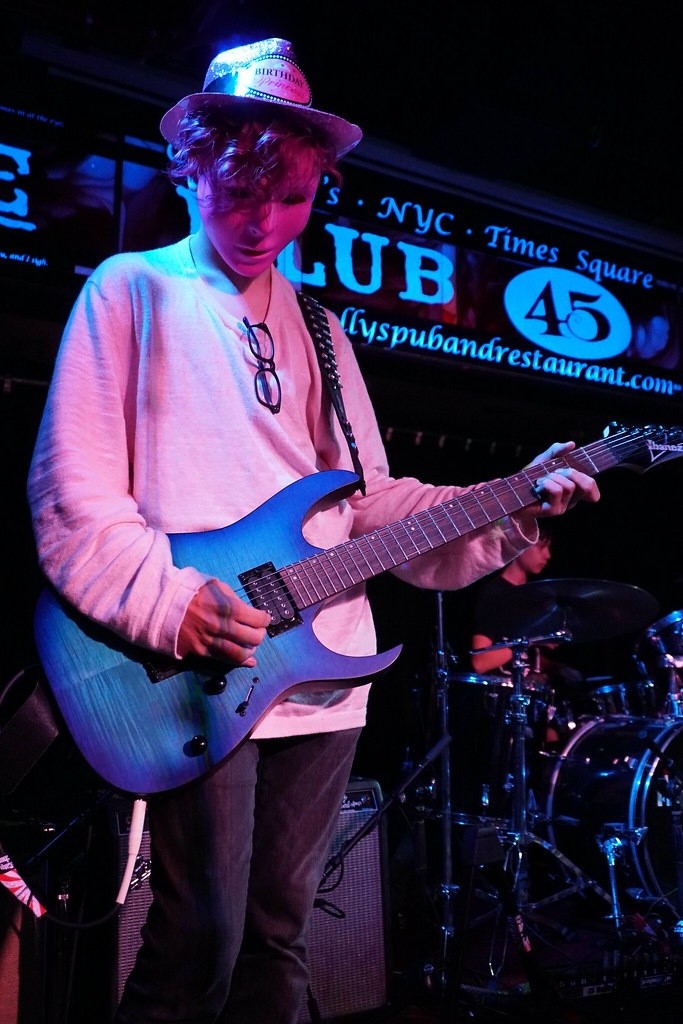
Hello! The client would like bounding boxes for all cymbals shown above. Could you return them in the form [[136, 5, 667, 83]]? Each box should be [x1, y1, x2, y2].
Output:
[[469, 577, 661, 646]]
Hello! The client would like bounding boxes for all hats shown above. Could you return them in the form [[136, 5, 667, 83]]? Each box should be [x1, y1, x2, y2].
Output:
[[160, 39, 363, 161]]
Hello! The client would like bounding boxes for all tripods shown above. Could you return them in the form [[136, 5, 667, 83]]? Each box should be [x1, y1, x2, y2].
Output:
[[376, 588, 661, 1010]]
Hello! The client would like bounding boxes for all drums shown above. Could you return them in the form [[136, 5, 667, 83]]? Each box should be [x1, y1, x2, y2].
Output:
[[383, 802, 538, 937], [551, 673, 665, 738], [630, 608, 683, 682], [544, 713, 683, 929], [420, 669, 558, 770]]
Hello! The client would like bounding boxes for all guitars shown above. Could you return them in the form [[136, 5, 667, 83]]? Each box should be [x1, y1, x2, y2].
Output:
[[30, 420, 683, 806]]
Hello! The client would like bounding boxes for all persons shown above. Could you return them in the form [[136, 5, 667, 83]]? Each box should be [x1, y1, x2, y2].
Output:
[[465, 518, 565, 744], [21, 28, 602, 1024]]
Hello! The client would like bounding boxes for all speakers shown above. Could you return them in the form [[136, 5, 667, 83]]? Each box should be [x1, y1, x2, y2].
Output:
[[113, 774, 393, 1024]]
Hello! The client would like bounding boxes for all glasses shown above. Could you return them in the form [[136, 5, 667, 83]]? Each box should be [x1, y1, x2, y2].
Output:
[[242, 316, 282, 415]]
